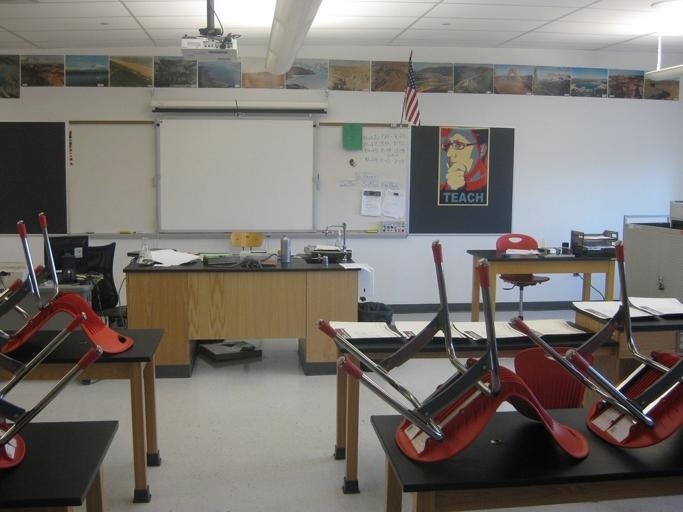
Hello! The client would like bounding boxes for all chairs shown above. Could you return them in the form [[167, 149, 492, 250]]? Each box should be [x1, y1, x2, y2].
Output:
[[230, 231, 263, 252], [319, 241, 591, 462], [507, 242, 682, 451], [497, 234, 552, 318], [0, 211, 134, 363]]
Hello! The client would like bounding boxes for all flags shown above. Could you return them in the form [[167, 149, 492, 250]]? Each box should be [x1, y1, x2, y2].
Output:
[[402, 56, 421, 128]]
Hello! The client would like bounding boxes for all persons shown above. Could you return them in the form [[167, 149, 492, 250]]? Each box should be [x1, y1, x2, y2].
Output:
[[438, 125, 490, 196]]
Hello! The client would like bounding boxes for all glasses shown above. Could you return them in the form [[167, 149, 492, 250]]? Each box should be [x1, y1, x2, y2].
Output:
[[442, 141, 474, 152]]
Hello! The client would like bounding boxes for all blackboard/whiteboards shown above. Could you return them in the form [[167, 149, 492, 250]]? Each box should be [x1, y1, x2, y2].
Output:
[[69, 120, 157, 234], [314, 123, 410, 236], [1, 121, 69, 234], [411, 126, 514, 236], [156, 117, 317, 234]]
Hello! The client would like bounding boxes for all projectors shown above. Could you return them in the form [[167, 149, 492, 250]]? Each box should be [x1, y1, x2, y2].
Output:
[[179, 35, 239, 64]]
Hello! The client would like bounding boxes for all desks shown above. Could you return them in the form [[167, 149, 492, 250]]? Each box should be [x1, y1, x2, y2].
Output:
[[0, 273, 164, 511], [569, 297, 681, 362], [371, 413, 681, 461], [327, 317, 621, 461], [123, 254, 360, 379], [465, 251, 618, 320]]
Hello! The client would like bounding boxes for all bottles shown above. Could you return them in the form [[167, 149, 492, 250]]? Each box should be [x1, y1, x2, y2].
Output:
[[139, 236, 153, 266], [264, 234, 273, 255], [280, 233, 291, 263]]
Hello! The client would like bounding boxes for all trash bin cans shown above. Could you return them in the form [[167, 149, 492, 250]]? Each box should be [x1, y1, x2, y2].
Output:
[[358, 302, 393, 372]]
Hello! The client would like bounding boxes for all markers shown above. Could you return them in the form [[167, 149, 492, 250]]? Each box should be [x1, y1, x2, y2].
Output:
[[364, 230, 378, 233], [84, 232, 94, 234], [120, 232, 131, 234]]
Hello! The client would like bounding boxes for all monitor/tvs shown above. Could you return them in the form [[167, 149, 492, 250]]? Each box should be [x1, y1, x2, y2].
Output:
[[44, 234, 89, 284]]
